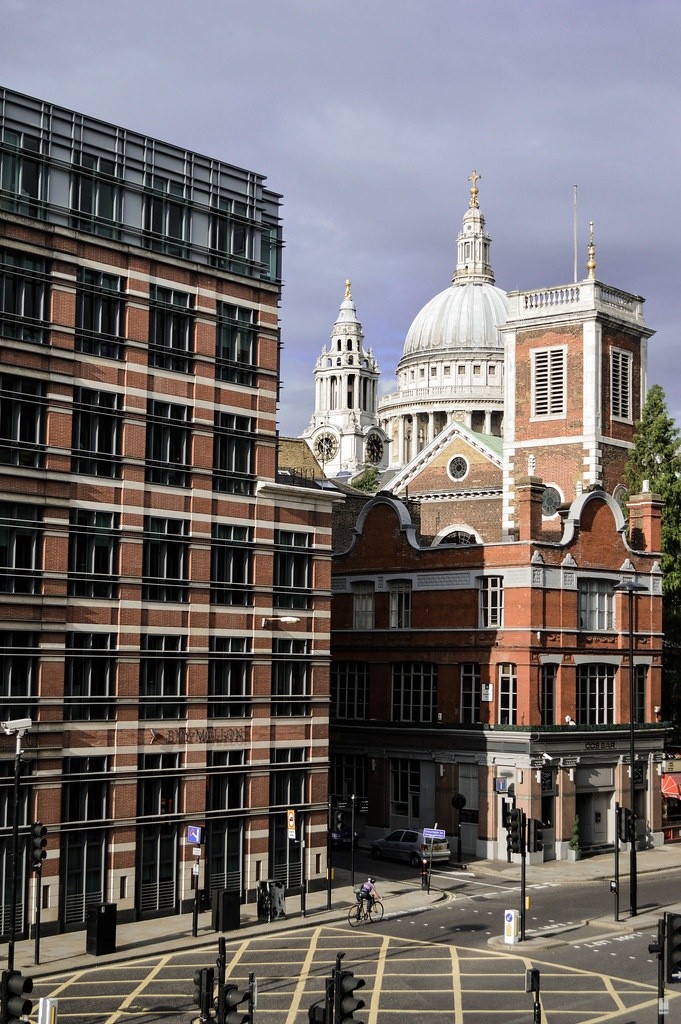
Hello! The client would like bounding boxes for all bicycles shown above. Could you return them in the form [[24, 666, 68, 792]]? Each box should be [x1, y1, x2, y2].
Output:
[[348, 895, 384, 928]]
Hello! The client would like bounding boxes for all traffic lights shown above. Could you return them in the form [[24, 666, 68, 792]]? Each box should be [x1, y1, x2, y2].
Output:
[[528, 819, 544, 853], [193, 967, 213, 1024], [223, 985, 251, 1024], [618, 807, 633, 842], [333, 810, 345, 833], [30, 822, 47, 863], [666, 913, 681, 983], [505, 809, 522, 854], [1, 970, 33, 1024], [335, 971, 365, 1024]]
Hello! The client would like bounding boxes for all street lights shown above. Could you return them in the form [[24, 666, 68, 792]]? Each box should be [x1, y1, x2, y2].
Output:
[[613, 581, 650, 918]]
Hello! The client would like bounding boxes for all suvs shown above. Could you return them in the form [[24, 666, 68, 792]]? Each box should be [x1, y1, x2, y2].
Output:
[[330, 827, 360, 852], [370, 829, 451, 868]]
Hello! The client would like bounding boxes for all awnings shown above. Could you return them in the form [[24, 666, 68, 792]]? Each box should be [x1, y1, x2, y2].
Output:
[[661, 773, 681, 801]]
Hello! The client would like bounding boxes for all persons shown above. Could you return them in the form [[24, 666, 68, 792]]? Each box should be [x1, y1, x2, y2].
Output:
[[355, 877, 381, 920]]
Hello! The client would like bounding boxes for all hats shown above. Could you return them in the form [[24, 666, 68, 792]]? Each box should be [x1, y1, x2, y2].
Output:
[[370, 878, 376, 883]]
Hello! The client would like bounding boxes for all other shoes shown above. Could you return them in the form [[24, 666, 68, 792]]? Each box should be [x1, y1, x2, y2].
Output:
[[367, 910, 372, 913]]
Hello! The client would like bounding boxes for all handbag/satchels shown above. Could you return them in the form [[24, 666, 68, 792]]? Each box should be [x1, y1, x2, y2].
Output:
[[353, 883, 363, 893]]
